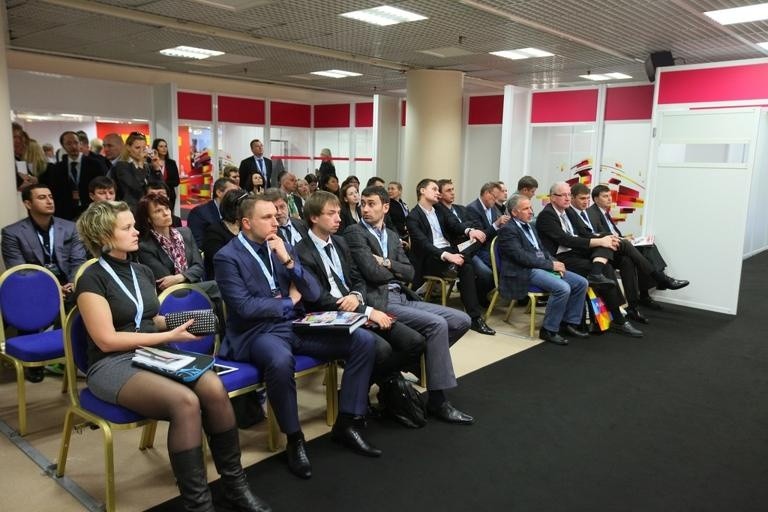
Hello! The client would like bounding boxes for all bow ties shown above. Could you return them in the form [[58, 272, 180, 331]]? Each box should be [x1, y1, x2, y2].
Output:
[[521, 223, 529, 229]]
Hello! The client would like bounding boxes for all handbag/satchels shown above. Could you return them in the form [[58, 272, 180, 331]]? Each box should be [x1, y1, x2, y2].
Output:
[[585, 287, 628, 332], [165, 309, 219, 336], [376, 376, 428, 429]]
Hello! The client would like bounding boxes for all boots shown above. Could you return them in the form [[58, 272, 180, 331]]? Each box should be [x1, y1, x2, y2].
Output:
[[207, 429, 273, 512], [169, 446, 215, 512]]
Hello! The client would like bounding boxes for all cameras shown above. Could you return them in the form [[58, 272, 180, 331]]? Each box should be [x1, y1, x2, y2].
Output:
[[144, 148, 154, 157]]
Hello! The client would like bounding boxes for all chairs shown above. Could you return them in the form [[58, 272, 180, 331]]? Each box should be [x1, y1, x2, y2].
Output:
[[273, 350, 334, 443], [72, 257, 121, 300], [1, 265, 92, 435], [150, 283, 278, 459], [415, 237, 548, 339], [54, 301, 184, 510]]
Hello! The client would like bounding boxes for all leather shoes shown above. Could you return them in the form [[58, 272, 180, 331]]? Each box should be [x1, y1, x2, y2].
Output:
[[441, 263, 458, 279], [426, 400, 474, 424], [610, 296, 659, 338], [656, 275, 689, 290], [540, 324, 590, 345], [331, 424, 383, 457], [24, 366, 45, 382], [470, 318, 495, 334], [536, 299, 547, 307], [286, 434, 312, 479], [588, 273, 616, 287]]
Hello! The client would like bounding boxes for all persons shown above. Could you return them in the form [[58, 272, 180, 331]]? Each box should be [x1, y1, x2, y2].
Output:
[[2, 125, 408, 382], [214, 182, 475, 476], [404, 176, 689, 345], [77, 200, 271, 511]]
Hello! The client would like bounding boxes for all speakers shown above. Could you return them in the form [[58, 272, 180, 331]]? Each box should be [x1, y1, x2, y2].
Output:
[[644, 50, 675, 83]]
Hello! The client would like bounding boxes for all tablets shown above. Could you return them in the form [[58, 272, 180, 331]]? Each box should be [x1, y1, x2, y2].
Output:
[[212, 362, 239, 375]]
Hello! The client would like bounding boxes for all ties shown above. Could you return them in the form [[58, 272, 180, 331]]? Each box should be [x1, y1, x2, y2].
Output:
[[560, 214, 572, 237], [289, 196, 296, 214], [280, 226, 297, 245], [324, 244, 349, 297], [581, 212, 589, 224], [257, 159, 264, 175], [71, 162, 77, 181], [605, 212, 622, 237]]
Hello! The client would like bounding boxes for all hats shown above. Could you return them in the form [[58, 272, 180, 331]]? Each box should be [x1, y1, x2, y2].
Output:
[[321, 149, 331, 157]]
